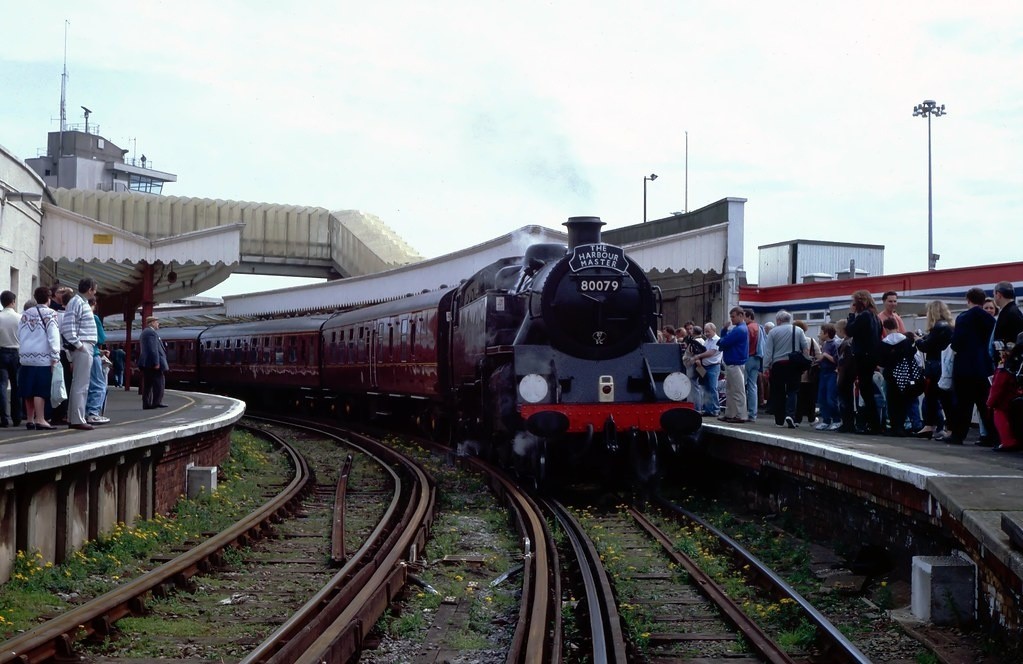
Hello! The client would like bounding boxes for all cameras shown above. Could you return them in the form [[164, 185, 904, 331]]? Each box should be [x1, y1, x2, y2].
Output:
[[913, 328, 926, 337]]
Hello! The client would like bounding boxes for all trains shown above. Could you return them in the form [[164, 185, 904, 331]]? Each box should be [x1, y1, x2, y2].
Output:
[[104, 216, 702, 489]]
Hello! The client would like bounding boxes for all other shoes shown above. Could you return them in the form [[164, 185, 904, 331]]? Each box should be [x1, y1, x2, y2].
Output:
[[26, 422, 35, 430], [142, 404, 168, 411], [696, 409, 757, 423], [13, 419, 20, 426], [69, 421, 93, 430], [86, 416, 110, 423], [35, 422, 57, 430], [0, 421, 8, 428], [51, 415, 70, 424], [760, 400, 767, 408], [776, 414, 1022, 452]]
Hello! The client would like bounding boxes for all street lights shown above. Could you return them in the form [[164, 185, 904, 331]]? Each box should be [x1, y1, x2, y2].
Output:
[[912, 99, 947, 270], [644, 173, 657, 224]]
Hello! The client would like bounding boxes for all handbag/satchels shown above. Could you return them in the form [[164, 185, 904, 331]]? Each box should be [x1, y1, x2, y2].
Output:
[[50, 359, 68, 408], [61, 333, 75, 351], [789, 351, 811, 372], [986, 367, 1017, 411], [835, 365, 849, 392], [938, 344, 957, 391]]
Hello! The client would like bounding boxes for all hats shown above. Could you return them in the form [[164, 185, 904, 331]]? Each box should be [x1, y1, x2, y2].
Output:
[[147, 315, 159, 324]]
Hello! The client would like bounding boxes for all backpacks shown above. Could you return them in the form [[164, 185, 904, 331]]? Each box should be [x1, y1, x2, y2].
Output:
[[893, 352, 926, 399], [690, 338, 707, 354]]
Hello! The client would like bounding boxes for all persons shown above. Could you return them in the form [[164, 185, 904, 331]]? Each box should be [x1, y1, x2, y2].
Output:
[[657, 306, 821, 429], [834, 289, 886, 435], [50, 287, 74, 425], [880, 316, 918, 437], [903, 332, 922, 431], [0, 290, 22, 426], [137, 316, 169, 409], [814, 323, 843, 430], [982, 297, 998, 317], [835, 319, 853, 369], [942, 288, 1000, 447], [62, 277, 98, 430], [877, 290, 906, 338], [986, 281, 1023, 452], [17, 287, 61, 430], [911, 300, 953, 440], [130, 344, 143, 394], [110, 343, 126, 388], [88, 296, 111, 423]]
